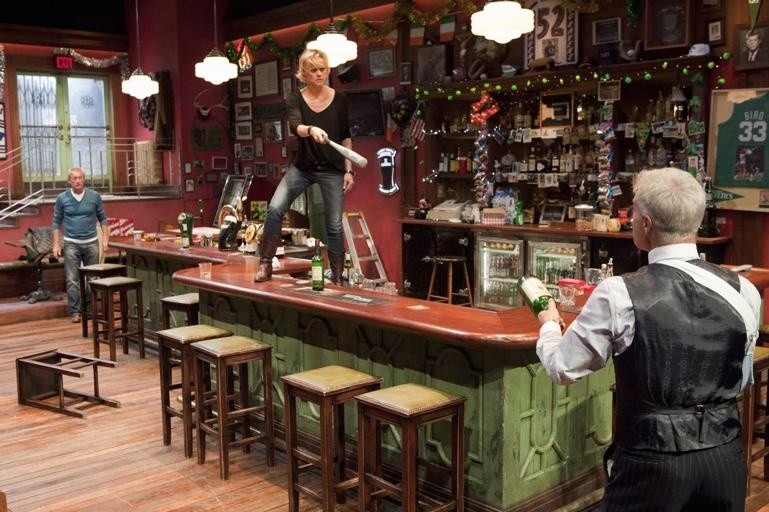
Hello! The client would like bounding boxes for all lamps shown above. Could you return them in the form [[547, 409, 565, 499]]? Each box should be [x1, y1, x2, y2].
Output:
[[121, 1, 160, 101], [470, 0, 534, 46], [194, 1, 238, 86], [307, 0, 359, 69]]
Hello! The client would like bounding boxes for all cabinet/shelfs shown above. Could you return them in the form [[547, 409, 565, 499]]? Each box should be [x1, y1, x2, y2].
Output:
[[589, 237, 648, 277], [401, 222, 475, 308], [414, 56, 711, 233]]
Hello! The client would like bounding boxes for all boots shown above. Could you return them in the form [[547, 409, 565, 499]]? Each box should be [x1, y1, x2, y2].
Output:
[[329, 255, 345, 285], [255, 233, 280, 282]]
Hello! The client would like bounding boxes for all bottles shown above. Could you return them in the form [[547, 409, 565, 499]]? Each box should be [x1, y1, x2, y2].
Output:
[[483, 253, 519, 306], [574, 204, 593, 232], [311, 239, 324, 291], [577, 93, 598, 124], [515, 273, 565, 334], [499, 100, 533, 128], [700, 174, 718, 237], [353, 266, 398, 296], [534, 256, 577, 299], [182, 223, 189, 249], [630, 80, 689, 124], [482, 187, 525, 226], [439, 149, 473, 173], [344, 251, 353, 281], [520, 142, 587, 173], [623, 135, 658, 171]]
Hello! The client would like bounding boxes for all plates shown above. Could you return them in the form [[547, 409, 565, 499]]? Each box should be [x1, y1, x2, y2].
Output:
[[244, 222, 264, 244]]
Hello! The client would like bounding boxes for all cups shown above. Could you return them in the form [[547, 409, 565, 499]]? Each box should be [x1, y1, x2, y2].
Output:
[[592, 214, 621, 232], [198, 262, 213, 278], [132, 230, 142, 241], [381, 156, 395, 190]]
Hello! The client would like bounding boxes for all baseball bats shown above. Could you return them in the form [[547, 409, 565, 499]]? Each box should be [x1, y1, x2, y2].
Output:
[[322, 134, 368, 169]]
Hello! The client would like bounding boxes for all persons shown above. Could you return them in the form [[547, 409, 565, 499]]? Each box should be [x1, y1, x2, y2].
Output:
[[535, 164, 764, 511], [51, 166, 110, 322], [253, 47, 356, 286]]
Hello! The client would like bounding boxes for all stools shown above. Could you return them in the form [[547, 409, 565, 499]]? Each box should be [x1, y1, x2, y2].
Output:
[[426, 257, 473, 306], [191, 337, 277, 480], [75, 263, 128, 341], [159, 294, 202, 382], [733, 344, 769, 499], [14, 346, 121, 419], [86, 277, 145, 362], [154, 324, 250, 458], [353, 381, 469, 511], [279, 364, 382, 511]]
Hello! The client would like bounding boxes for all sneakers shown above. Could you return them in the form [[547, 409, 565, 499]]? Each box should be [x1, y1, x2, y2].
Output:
[[72, 313, 80, 322]]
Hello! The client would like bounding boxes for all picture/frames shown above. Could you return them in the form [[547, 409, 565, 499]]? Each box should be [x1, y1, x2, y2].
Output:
[[211, 173, 255, 228], [591, 18, 621, 46], [704, 17, 726, 48], [365, 46, 398, 80], [540, 205, 567, 223], [731, 19, 769, 73], [232, 48, 332, 179], [644, 0, 689, 50], [184, 155, 230, 193]]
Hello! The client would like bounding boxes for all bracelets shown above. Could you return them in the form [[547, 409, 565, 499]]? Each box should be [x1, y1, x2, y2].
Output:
[[344, 167, 356, 176], [307, 125, 315, 136]]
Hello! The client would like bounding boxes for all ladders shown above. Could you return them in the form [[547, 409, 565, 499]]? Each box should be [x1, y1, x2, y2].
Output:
[[343, 211, 389, 282]]
